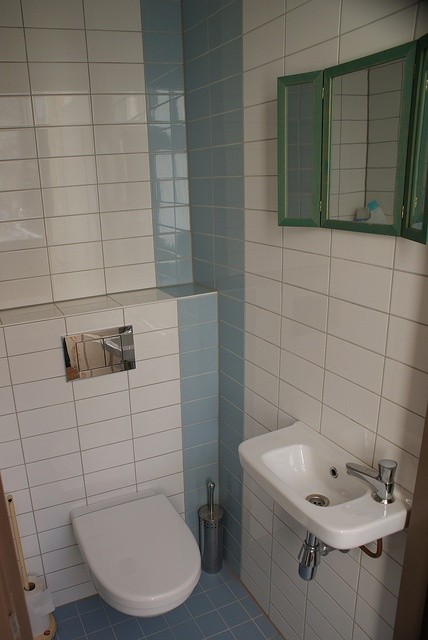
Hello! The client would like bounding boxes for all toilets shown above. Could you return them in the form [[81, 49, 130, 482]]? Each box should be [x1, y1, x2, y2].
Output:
[[70, 490, 202, 616]]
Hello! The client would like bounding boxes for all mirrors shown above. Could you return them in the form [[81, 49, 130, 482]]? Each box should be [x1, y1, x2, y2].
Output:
[[277, 33, 428, 245]]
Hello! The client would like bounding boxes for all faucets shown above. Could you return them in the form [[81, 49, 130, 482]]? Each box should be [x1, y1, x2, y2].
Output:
[[343, 458, 405, 507]]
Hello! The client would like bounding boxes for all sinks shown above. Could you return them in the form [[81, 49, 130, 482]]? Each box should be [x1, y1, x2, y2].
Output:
[[236, 422, 406, 549]]
